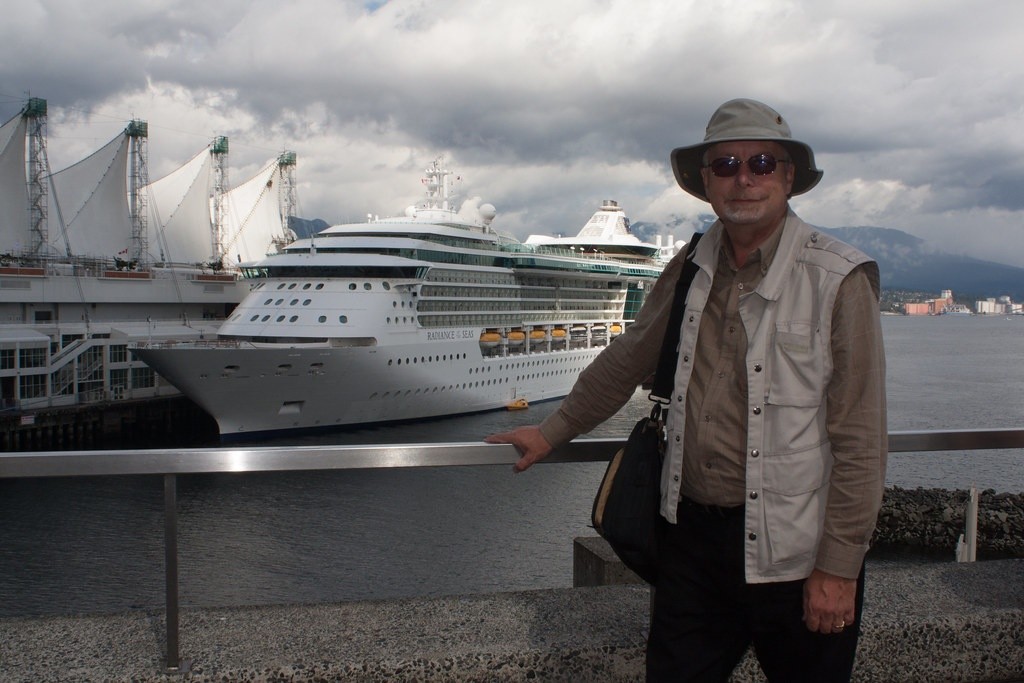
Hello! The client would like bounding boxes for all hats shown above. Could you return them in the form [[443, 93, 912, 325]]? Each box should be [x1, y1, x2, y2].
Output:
[[670, 98, 824, 203]]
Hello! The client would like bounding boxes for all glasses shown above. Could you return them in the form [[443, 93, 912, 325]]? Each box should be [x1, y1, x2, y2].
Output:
[[703, 153, 789, 176]]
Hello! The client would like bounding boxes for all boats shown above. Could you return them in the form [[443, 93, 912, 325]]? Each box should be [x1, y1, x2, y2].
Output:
[[610, 326, 622, 338], [505, 399, 528, 411], [590, 326, 606, 341], [551, 330, 566, 342], [128, 157, 687, 444], [480, 333, 502, 348], [506, 332, 526, 345], [570, 327, 587, 343], [530, 330, 546, 343]]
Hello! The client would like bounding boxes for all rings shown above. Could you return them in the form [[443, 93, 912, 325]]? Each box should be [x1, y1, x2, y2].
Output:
[[833, 621, 845, 629]]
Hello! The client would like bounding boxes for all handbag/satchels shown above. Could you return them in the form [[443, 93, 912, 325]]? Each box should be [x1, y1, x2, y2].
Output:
[[591, 418, 667, 583]]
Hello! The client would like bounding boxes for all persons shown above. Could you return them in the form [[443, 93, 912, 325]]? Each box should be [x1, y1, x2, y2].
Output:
[[484, 98, 889, 683]]
